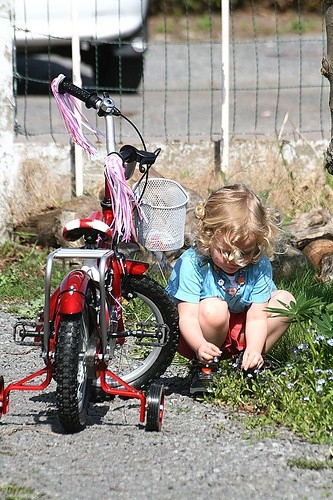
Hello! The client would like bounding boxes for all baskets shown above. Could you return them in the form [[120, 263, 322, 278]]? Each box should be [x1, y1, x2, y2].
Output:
[[133, 178, 189, 252]]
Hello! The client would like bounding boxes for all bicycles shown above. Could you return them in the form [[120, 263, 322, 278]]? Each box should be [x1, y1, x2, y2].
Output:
[[0, 74, 190, 431]]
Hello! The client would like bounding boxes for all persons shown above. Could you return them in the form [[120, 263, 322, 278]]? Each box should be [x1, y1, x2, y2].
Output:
[[165, 184, 297, 395]]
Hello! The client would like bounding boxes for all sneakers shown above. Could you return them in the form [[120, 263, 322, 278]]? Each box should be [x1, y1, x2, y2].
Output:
[[191, 365, 218, 396]]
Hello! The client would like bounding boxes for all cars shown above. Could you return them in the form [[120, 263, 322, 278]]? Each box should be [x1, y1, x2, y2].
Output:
[[15, 0, 151, 96]]
